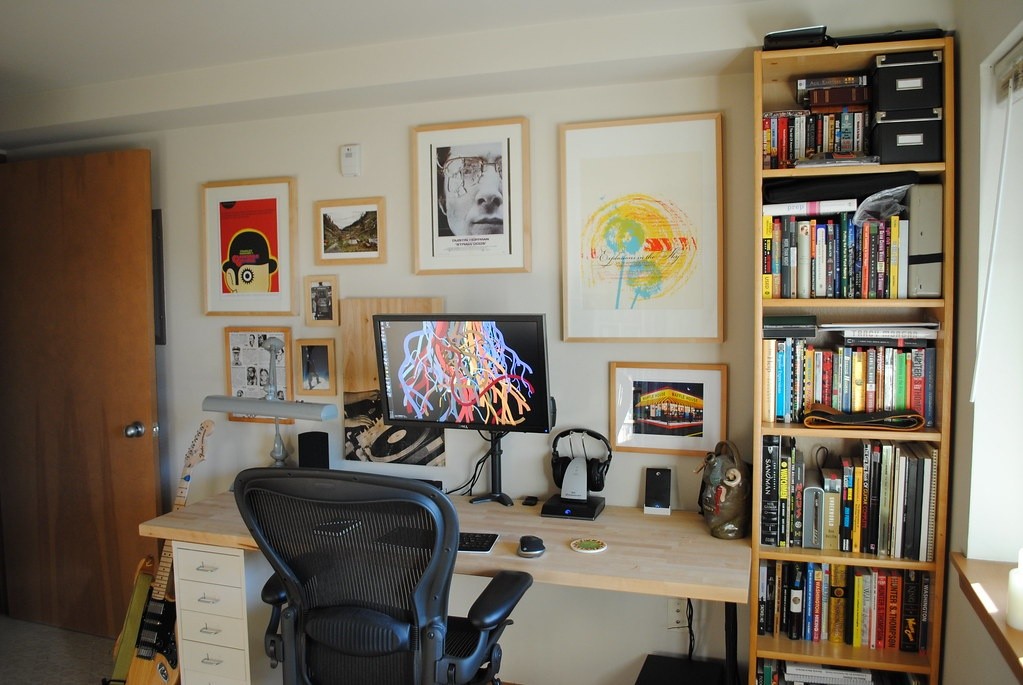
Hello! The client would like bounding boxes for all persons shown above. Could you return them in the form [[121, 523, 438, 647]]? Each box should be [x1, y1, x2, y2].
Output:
[[247, 334, 257, 347], [231, 347, 243, 367], [442, 144, 504, 236], [247, 367, 269, 386], [304, 353, 321, 390], [344, 389, 380, 429]]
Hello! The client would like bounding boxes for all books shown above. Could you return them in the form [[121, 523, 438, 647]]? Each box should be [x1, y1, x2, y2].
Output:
[[763, 75, 874, 170], [763, 436, 939, 563], [762, 199, 910, 299], [756, 660, 928, 685], [763, 314, 941, 427], [758, 560, 932, 655]]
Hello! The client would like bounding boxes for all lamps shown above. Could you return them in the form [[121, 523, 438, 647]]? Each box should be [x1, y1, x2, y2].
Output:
[[202, 394, 338, 469]]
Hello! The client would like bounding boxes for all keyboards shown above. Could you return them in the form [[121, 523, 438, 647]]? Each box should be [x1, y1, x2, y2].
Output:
[[375, 526, 500, 553]]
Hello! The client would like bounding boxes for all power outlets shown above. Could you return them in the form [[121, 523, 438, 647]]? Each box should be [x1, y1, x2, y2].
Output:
[[667, 599, 688, 629]]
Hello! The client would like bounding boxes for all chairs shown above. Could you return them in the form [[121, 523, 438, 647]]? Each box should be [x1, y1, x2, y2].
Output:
[[231, 467, 531, 685]]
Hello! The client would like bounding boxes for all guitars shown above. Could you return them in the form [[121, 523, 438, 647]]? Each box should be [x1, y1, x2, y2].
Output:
[[114, 420, 217, 684]]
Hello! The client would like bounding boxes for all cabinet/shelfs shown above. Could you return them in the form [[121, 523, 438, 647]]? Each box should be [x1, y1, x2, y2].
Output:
[[171, 539, 287, 685], [751, 30, 958, 685]]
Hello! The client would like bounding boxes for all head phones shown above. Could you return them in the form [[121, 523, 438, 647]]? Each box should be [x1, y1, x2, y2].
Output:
[[551, 428, 612, 491]]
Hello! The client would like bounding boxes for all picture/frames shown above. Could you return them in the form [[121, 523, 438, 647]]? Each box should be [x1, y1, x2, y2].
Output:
[[304, 272, 339, 325], [609, 361, 729, 454], [558, 111, 726, 342], [295, 338, 336, 396], [313, 197, 388, 263], [199, 178, 301, 313], [408, 116, 530, 275], [224, 326, 293, 424]]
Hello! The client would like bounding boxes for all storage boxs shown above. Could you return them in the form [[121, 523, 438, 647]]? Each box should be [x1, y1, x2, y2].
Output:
[[797, 75, 868, 109], [870, 50, 941, 111], [869, 113, 940, 164]]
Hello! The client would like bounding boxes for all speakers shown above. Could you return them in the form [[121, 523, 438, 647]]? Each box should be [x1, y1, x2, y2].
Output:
[[298, 431, 329, 469], [645, 468, 671, 508]]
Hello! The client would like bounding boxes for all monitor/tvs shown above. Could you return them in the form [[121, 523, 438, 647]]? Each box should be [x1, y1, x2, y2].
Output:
[[372, 314, 552, 506]]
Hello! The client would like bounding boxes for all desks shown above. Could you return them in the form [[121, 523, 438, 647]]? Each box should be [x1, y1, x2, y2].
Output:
[[138, 490, 754, 685]]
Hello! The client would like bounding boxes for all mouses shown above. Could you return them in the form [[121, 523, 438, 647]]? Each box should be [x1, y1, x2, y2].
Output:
[[517, 535, 546, 557]]
